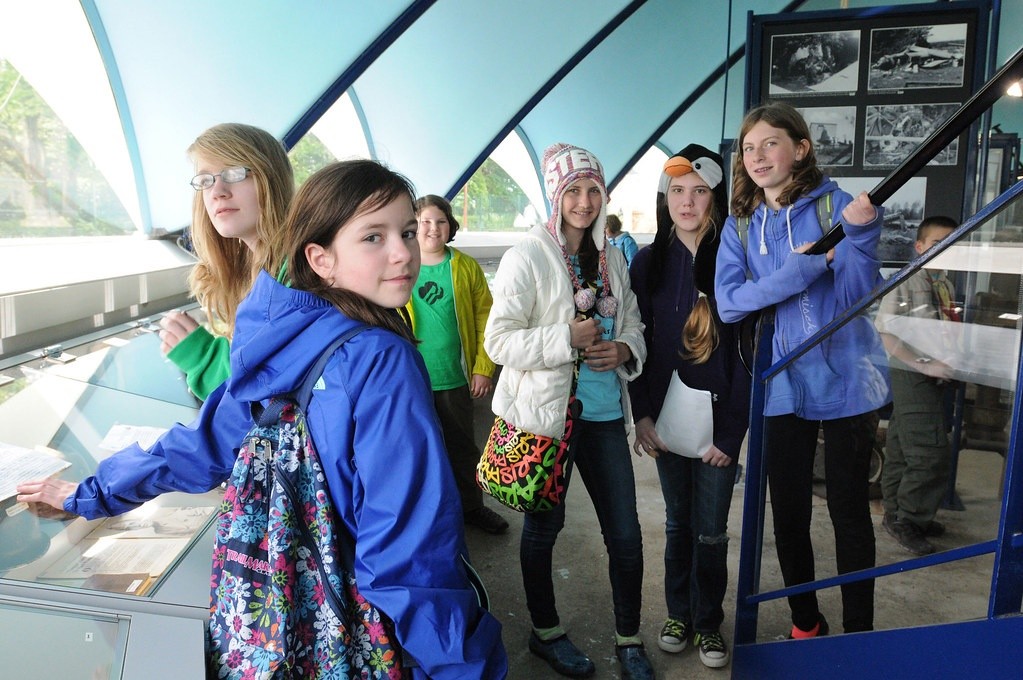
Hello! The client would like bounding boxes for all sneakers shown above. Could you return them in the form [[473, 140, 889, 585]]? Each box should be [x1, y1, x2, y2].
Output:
[[694, 632, 728, 667], [658, 617, 687, 652]]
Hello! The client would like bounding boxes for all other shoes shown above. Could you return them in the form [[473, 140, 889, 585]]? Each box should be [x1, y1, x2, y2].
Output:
[[787, 611, 829, 640], [882, 512, 945, 555]]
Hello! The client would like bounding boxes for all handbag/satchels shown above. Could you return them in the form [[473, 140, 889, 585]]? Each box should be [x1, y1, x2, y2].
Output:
[[477, 408, 571, 512]]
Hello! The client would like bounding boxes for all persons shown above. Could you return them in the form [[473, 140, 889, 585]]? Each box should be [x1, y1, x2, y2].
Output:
[[157, 121, 303, 404], [16, 159, 511, 680], [873, 215, 965, 557], [394, 193, 510, 534], [482, 144, 759, 680], [604, 214, 639, 270], [714, 103, 894, 641]]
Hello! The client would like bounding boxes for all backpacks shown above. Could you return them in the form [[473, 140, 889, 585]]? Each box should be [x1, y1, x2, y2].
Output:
[[211, 325, 405, 680]]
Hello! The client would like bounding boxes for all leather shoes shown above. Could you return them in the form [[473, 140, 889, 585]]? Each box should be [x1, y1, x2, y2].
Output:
[[529, 628, 595, 678], [615, 643, 655, 680]]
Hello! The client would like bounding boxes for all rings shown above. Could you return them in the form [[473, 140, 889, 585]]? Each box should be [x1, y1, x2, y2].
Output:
[[180, 310, 186, 315]]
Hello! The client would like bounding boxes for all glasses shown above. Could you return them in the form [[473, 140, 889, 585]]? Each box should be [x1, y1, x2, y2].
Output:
[[190, 166, 251, 191]]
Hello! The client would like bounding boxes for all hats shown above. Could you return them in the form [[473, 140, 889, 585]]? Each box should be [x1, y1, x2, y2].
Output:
[[540, 144, 618, 317], [644, 144, 727, 295]]
